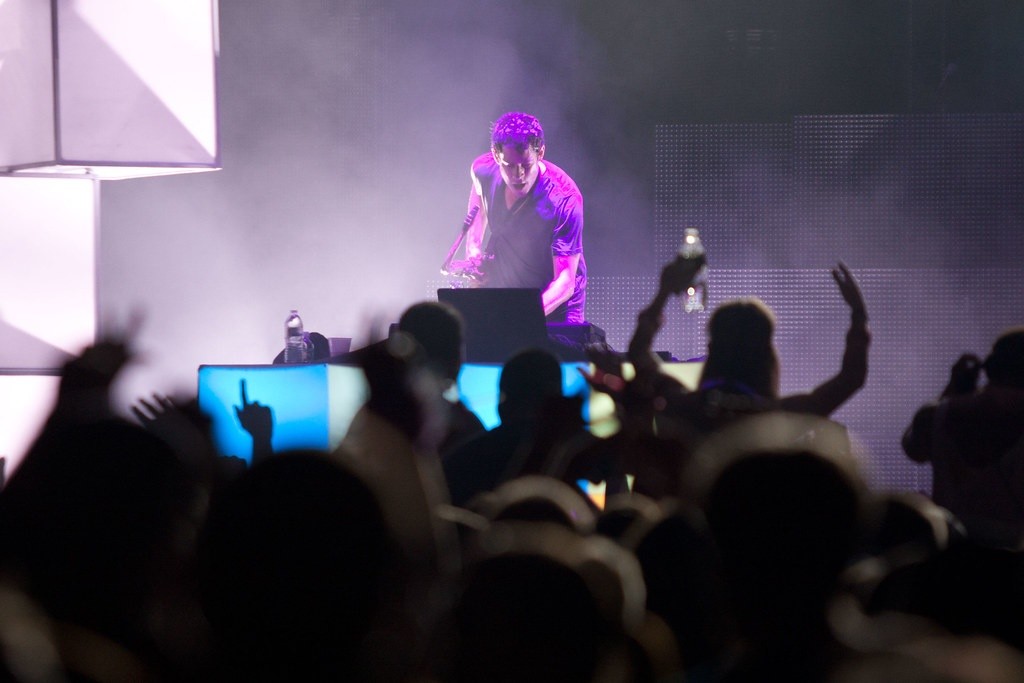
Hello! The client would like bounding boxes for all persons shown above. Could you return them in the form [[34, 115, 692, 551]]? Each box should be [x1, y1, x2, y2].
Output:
[[0, 255, 1024, 683], [465, 113, 587, 323]]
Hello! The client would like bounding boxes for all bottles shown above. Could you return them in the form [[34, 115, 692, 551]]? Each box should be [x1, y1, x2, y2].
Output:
[[676, 228, 708, 312], [284, 311, 314, 364]]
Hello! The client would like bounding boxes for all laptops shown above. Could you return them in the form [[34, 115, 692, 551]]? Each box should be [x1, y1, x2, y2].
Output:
[[438, 286, 550, 366]]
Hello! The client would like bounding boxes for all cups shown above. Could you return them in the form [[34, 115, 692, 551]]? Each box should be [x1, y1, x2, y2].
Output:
[[328, 337, 351, 358]]
